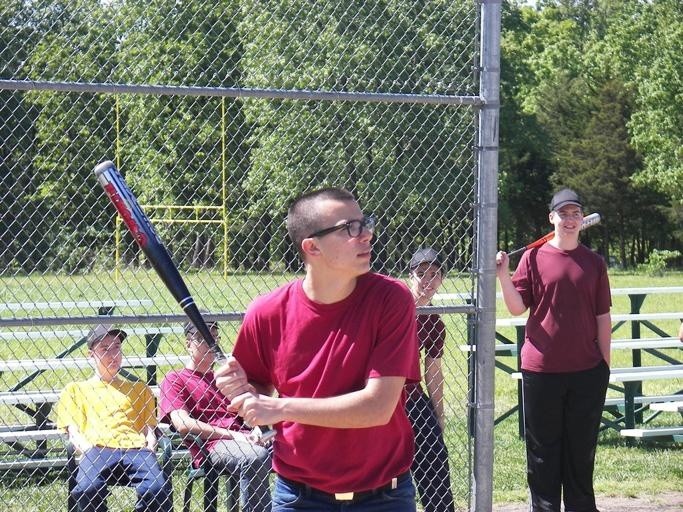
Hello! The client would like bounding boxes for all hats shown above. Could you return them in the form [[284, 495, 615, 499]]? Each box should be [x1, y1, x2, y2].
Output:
[[88, 324, 127, 350], [549, 188, 581, 209], [183, 311, 220, 334], [410, 249, 443, 270]]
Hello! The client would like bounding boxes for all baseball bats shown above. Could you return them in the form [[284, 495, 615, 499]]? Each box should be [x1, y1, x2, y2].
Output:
[[93, 159, 276, 443], [507, 213, 601, 256]]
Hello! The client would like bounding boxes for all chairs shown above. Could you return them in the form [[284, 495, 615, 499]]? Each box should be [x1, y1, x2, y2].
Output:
[[179, 431, 279, 512], [66, 428, 173, 512]]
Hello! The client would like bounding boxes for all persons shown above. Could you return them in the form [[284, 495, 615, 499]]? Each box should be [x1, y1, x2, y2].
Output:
[[494, 188, 613, 512], [56, 322, 166, 512], [401, 248, 455, 511], [159, 309, 275, 511], [213, 187, 422, 512]]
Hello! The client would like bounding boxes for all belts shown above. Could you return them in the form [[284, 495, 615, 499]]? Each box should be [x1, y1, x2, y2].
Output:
[[277, 470, 410, 501]]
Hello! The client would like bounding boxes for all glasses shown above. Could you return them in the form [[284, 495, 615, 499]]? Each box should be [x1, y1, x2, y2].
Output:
[[308, 217, 376, 237]]
